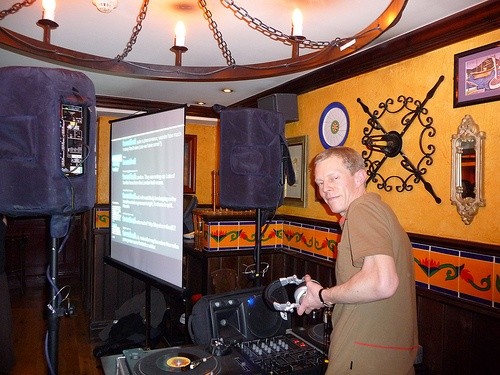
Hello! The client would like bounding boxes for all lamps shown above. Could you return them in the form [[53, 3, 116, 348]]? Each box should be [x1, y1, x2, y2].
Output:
[[356, 74, 445, 204], [0, 1, 409, 83]]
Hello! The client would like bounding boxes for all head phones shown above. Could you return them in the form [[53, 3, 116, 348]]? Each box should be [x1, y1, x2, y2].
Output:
[[265, 275, 322, 312]]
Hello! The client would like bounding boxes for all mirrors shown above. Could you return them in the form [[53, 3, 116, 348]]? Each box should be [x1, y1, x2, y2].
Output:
[[449, 114, 487, 225]]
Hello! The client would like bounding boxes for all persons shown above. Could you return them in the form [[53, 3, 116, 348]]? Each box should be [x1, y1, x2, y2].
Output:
[[297, 147, 417, 375]]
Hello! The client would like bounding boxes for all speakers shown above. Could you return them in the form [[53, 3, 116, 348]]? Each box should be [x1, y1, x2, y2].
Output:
[[0, 65, 96, 217], [220, 109, 282, 210], [191, 285, 292, 347], [257, 93, 298, 123]]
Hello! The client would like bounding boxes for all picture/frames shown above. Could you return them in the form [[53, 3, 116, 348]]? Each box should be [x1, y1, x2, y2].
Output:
[[183, 134, 197, 194], [452, 41, 500, 109]]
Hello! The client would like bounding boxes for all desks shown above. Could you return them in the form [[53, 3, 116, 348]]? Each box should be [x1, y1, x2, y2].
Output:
[[5, 236, 28, 296], [99, 326, 328, 375]]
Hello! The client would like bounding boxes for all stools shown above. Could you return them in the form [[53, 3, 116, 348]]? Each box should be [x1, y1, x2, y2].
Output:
[[43, 254, 76, 319]]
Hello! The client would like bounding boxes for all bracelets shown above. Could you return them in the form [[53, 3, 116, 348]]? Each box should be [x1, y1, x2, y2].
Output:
[[319, 289, 329, 306]]
